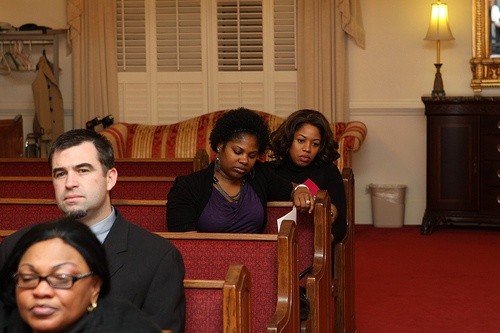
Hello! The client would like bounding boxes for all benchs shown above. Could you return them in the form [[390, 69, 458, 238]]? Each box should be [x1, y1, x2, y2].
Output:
[[1, 149, 209, 178], [0, 219, 300, 333], [0, 168, 355, 333], [183, 263, 251, 333], [0, 190, 330, 332]]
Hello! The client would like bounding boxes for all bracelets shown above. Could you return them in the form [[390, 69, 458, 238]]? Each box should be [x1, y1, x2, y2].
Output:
[[290, 184, 310, 192]]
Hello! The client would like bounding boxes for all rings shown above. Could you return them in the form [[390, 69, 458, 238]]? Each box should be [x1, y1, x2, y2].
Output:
[[330, 211, 335, 216], [306, 200, 311, 205]]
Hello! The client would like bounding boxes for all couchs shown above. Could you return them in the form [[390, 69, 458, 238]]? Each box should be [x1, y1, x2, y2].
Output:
[[97, 108, 367, 174]]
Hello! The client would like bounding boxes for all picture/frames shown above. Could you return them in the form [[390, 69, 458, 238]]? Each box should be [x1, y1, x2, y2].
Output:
[[469, 0, 500, 93]]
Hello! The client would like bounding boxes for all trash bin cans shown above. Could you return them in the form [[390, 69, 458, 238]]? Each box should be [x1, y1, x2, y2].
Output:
[[366, 183, 408, 229]]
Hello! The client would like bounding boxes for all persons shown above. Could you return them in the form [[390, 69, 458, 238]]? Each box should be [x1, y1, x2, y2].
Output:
[[166, 105, 314, 238], [0, 130, 187, 331], [1, 220, 162, 331], [257, 109, 349, 250]]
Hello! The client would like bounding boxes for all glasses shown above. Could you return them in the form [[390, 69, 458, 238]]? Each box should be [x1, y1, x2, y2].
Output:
[[12, 272, 95, 289]]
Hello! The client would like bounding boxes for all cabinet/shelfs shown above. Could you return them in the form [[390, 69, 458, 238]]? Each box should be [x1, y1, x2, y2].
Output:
[[420, 95, 500, 235]]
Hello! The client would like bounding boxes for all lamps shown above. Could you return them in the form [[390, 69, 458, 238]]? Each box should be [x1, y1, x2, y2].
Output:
[[423, 0, 456, 97]]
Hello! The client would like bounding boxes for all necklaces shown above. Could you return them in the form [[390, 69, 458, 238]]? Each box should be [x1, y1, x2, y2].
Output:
[[213, 176, 243, 204]]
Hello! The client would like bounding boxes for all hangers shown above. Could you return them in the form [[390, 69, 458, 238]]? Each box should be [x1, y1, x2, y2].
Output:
[[0, 40, 37, 75]]
